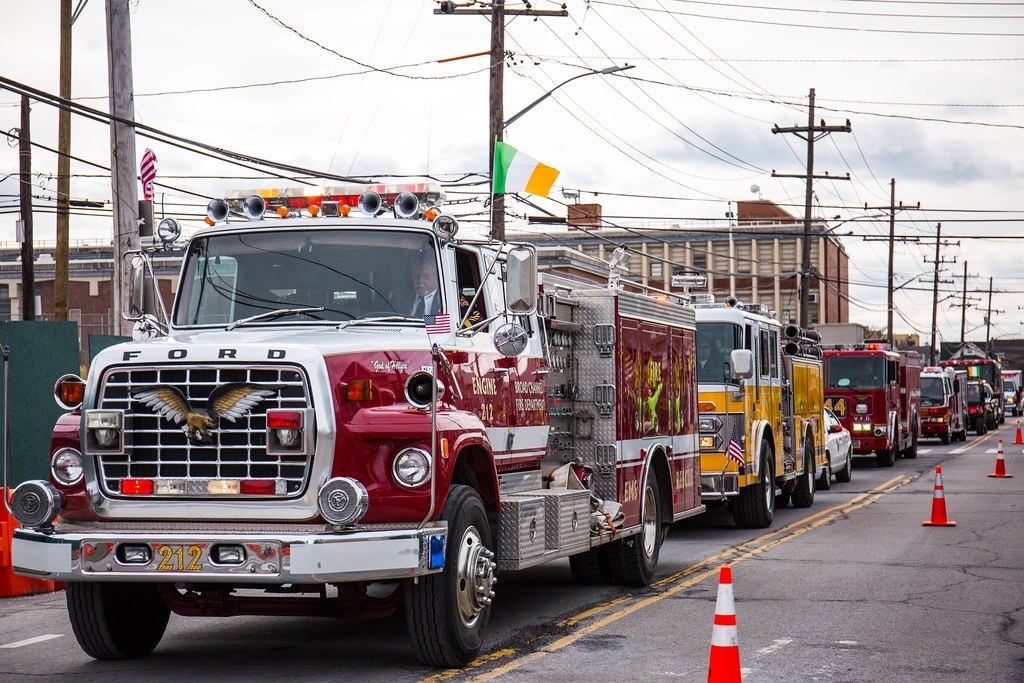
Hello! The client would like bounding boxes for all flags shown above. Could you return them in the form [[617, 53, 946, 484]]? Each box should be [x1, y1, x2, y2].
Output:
[[139, 148, 160, 206], [423, 313, 451, 334], [724, 426, 746, 468], [494, 140, 561, 198]]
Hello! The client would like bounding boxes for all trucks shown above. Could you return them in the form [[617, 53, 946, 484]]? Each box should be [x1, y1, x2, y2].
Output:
[[1000, 369, 1022, 416]]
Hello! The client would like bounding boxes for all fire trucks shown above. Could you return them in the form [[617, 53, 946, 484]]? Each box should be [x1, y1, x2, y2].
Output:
[[918, 366, 967, 444], [670, 269, 828, 529], [938, 358, 1005, 433], [10, 182, 706, 671], [818, 343, 921, 466]]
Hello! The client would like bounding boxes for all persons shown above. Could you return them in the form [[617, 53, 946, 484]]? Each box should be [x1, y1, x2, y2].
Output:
[[379, 248, 484, 330]]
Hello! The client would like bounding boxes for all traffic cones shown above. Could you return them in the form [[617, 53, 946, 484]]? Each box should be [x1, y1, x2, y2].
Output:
[[986, 439, 1014, 477], [922, 466, 956, 526], [706, 567, 742, 683], [1012, 420, 1024, 444]]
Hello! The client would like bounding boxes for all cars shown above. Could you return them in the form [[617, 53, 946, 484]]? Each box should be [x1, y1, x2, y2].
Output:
[[815, 406, 853, 490], [966, 380, 1002, 429]]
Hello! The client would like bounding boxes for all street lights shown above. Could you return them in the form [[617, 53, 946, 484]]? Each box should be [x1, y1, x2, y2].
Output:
[[489, 63, 637, 239], [800, 215, 886, 328], [888, 268, 949, 349], [986, 333, 1021, 358]]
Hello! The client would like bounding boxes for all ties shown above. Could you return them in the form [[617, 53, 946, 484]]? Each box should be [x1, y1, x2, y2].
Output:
[[414, 295, 425, 319]]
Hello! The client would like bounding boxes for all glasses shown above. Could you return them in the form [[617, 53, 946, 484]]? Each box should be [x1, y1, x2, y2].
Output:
[[699, 345, 709, 349]]
